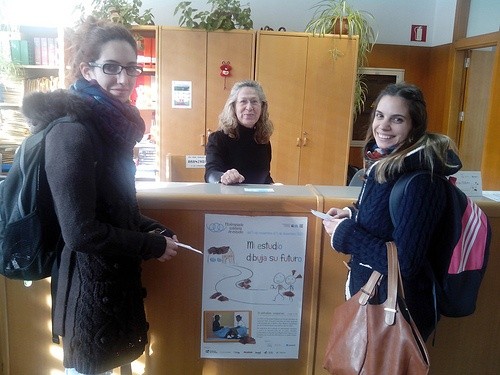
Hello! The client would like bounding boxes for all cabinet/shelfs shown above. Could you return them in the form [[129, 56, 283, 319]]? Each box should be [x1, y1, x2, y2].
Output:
[[0, 25, 360, 186]]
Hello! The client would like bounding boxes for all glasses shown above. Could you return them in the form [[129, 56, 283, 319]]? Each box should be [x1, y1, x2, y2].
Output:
[[88, 62, 143, 77]]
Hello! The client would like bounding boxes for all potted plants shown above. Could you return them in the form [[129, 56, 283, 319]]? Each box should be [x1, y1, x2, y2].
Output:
[[175, 0, 253, 31], [78, 0, 156, 27], [304, 0, 379, 123]]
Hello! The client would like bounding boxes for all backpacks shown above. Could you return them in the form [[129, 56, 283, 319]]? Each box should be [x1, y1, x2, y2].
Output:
[[1, 114, 106, 281], [390, 169, 493, 317]]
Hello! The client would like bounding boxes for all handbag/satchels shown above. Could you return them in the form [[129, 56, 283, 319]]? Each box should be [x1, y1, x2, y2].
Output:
[[323, 243, 431, 374]]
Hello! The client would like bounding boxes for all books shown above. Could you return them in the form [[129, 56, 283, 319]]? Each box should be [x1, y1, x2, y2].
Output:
[[9, 36, 59, 65], [0, 75, 60, 165]]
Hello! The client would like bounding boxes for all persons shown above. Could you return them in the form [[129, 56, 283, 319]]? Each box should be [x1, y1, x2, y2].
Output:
[[20, 18, 178, 375], [323, 82, 464, 344], [205, 81, 275, 184]]
